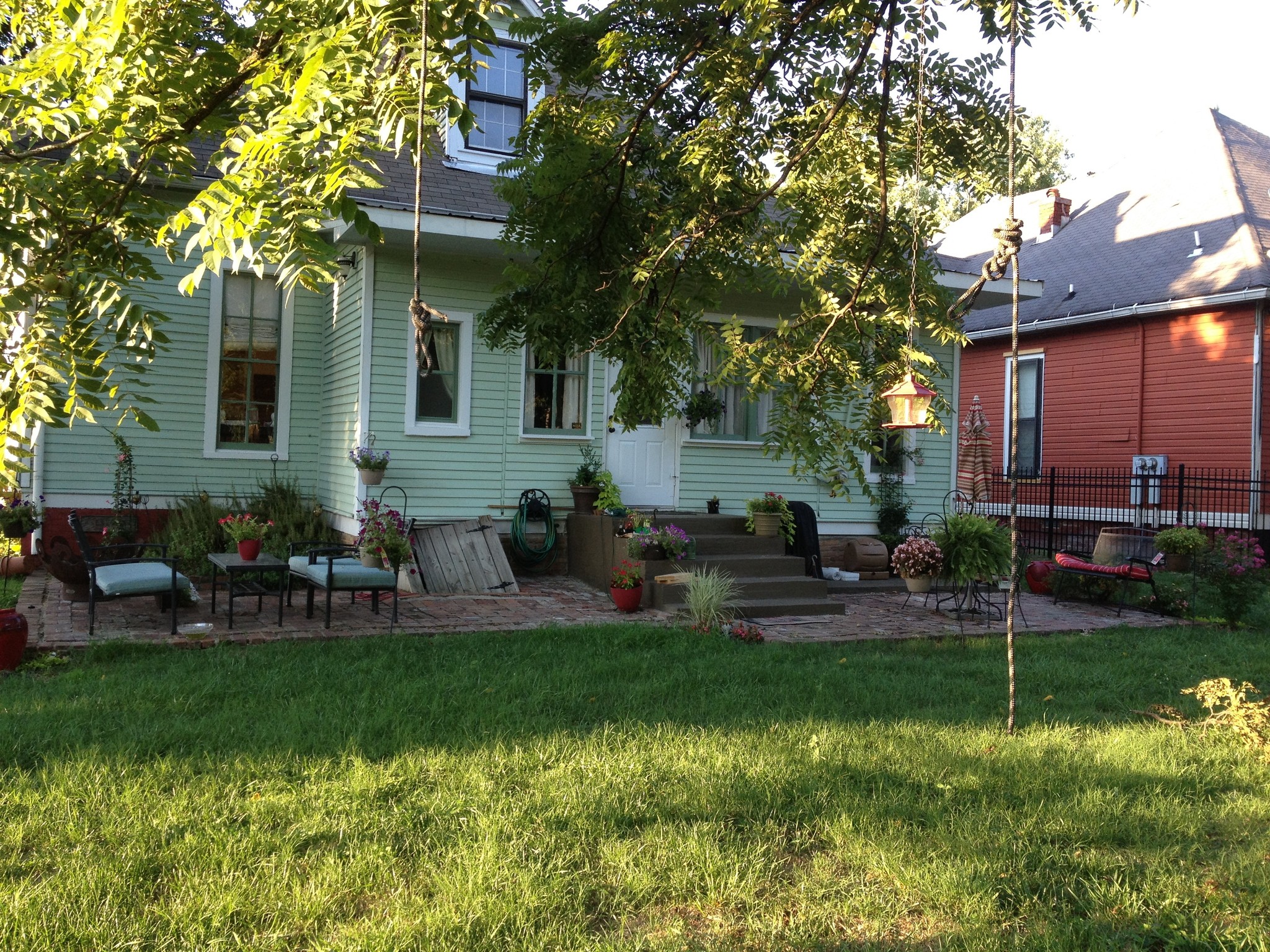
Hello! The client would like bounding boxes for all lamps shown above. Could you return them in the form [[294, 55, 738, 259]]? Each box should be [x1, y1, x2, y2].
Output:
[[880, 0, 938, 431]]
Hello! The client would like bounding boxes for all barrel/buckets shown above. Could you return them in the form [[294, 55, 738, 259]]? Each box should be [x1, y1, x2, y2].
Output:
[[0, 556, 34, 576]]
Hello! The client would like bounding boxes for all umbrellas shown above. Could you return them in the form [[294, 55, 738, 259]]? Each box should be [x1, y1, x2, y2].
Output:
[[957, 396, 993, 501]]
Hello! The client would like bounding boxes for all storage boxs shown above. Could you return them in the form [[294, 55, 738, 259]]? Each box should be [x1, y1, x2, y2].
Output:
[[838, 571, 860, 580]]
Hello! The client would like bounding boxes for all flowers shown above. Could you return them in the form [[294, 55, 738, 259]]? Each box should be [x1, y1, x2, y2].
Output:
[[184, 447, 423, 601], [0, 494, 46, 533], [609, 388, 944, 590]]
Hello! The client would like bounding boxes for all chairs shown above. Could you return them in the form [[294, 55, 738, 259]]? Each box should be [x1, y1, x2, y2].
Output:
[[286, 510, 416, 629], [971, 538, 1031, 628], [68, 510, 190, 635]]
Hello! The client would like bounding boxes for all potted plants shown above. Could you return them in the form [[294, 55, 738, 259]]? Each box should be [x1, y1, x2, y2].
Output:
[[1154, 527, 1209, 572], [707, 495, 719, 514], [567, 443, 625, 516]]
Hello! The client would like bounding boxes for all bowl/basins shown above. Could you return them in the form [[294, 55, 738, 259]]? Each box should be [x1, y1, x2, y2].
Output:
[[177, 623, 214, 639]]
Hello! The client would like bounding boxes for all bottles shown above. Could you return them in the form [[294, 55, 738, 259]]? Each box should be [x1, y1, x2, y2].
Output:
[[0, 608, 28, 670], [1025, 561, 1056, 595]]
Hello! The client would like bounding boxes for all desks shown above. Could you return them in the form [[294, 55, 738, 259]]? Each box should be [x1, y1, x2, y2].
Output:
[[207, 553, 290, 630]]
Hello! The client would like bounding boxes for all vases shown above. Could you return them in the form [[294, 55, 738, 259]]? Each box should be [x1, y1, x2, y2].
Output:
[[360, 547, 384, 569], [0, 607, 28, 670], [633, 526, 642, 535], [611, 578, 643, 613], [903, 573, 933, 592], [3, 520, 27, 537], [1025, 561, 1058, 595], [752, 512, 782, 537], [360, 468, 384, 485], [644, 544, 663, 560], [237, 540, 262, 561]]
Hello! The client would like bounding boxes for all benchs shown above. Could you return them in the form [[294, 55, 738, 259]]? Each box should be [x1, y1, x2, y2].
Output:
[[1054, 527, 1168, 618]]
